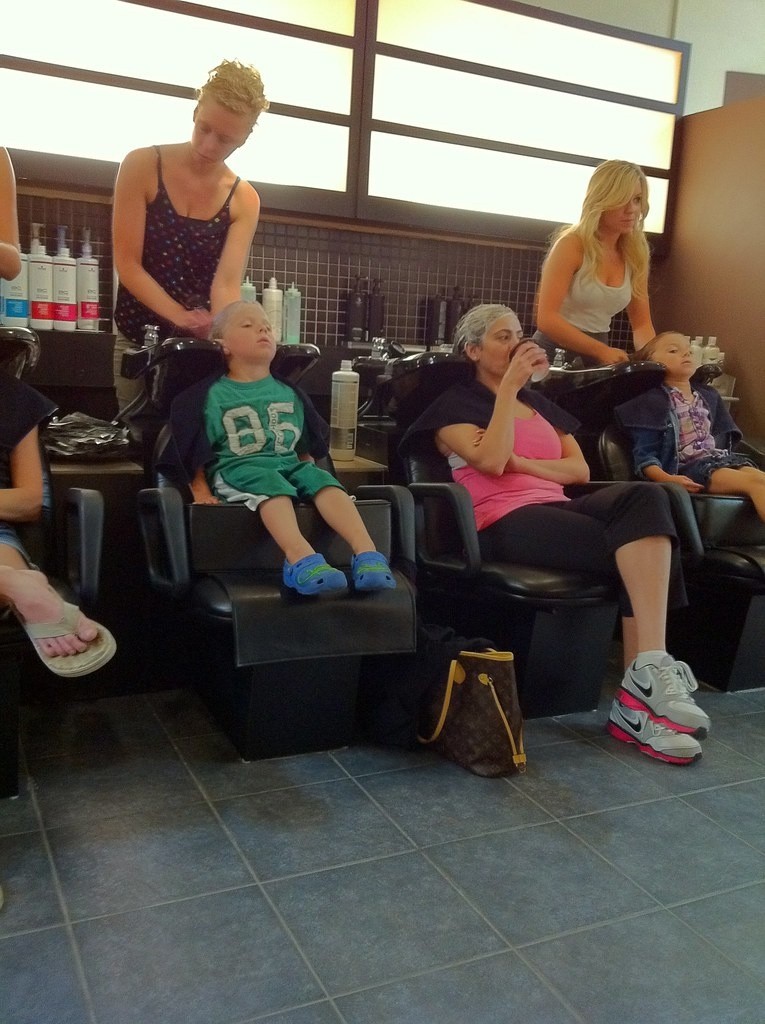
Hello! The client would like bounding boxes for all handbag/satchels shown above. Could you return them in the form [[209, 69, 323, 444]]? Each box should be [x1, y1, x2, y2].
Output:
[[419, 647, 527, 777]]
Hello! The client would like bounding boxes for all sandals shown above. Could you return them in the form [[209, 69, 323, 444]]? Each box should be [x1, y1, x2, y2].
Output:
[[351, 551, 396, 591], [283, 552, 348, 595]]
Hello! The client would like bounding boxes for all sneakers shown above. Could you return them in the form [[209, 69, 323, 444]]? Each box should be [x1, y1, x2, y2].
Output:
[[607, 698, 702, 764], [615, 655, 710, 740]]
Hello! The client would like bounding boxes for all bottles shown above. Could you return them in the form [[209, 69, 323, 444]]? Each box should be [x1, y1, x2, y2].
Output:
[[328, 361, 360, 460]]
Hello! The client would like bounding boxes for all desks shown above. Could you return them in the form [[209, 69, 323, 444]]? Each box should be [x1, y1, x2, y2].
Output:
[[333, 454, 389, 490], [49, 460, 143, 479]]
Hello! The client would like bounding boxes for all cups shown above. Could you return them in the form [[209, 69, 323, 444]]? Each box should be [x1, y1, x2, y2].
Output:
[[509, 339, 550, 382]]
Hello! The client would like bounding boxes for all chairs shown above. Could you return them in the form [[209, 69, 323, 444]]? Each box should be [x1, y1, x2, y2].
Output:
[[135, 429, 419, 763], [595, 425, 765, 695], [390, 434, 619, 721], [0, 439, 105, 799]]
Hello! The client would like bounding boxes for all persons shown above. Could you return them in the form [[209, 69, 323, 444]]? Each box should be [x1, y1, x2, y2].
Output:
[[0, 146, 22, 280], [431, 303, 710, 766], [628, 329, 765, 516], [112, 62, 267, 444], [0, 371, 115, 677], [533, 159, 655, 371], [168, 300, 398, 594]]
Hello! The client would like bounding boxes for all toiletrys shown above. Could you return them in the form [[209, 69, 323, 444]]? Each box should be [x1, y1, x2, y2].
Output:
[[240, 275, 302, 344], [428, 285, 479, 344], [327, 360, 360, 461], [346, 273, 386, 342], [1, 222, 99, 331], [685, 335, 729, 375]]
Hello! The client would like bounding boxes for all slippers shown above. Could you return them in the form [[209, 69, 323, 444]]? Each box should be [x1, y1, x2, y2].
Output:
[[8, 572, 117, 677]]
